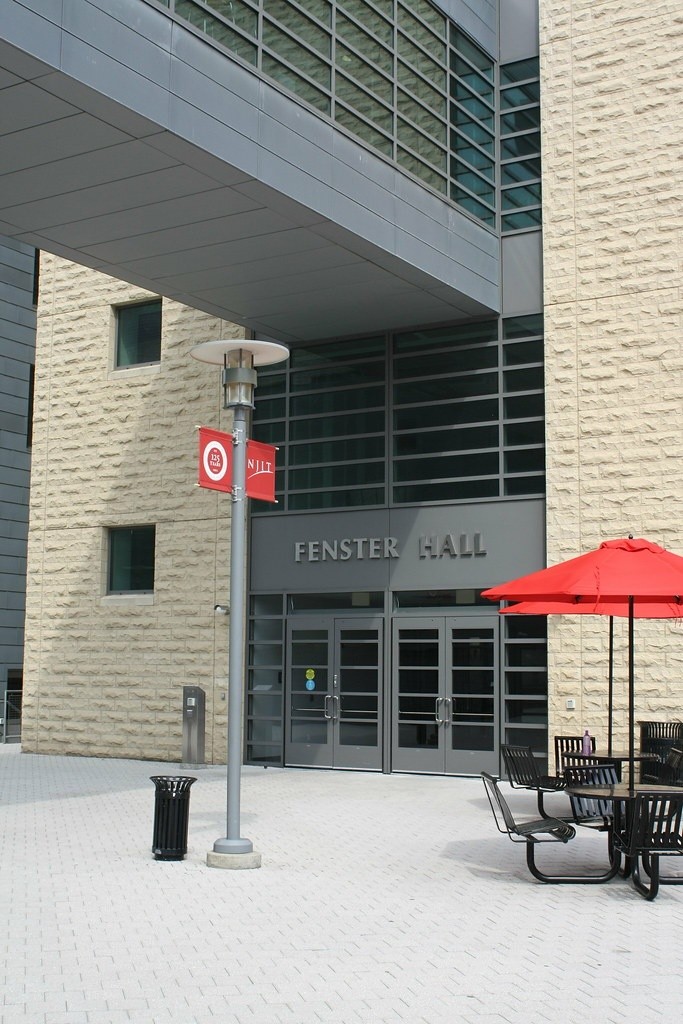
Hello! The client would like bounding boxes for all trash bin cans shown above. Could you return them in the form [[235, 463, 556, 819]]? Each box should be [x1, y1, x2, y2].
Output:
[[149, 775, 198, 861], [636, 720, 683, 785]]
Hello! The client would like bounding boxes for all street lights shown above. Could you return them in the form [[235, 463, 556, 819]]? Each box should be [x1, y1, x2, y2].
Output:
[[189, 339, 289, 869]]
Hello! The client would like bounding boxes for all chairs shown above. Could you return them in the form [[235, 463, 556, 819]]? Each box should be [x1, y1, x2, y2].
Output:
[[478, 722, 683, 899]]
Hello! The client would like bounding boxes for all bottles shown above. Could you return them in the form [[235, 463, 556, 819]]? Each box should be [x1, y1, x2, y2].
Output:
[[583, 730, 591, 756]]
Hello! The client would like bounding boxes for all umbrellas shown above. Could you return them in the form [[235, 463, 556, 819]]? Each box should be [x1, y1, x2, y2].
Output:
[[480, 534, 683, 797]]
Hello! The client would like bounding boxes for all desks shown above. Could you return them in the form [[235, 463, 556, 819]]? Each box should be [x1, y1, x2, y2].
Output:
[[562, 751, 659, 829], [565, 780, 683, 878]]
